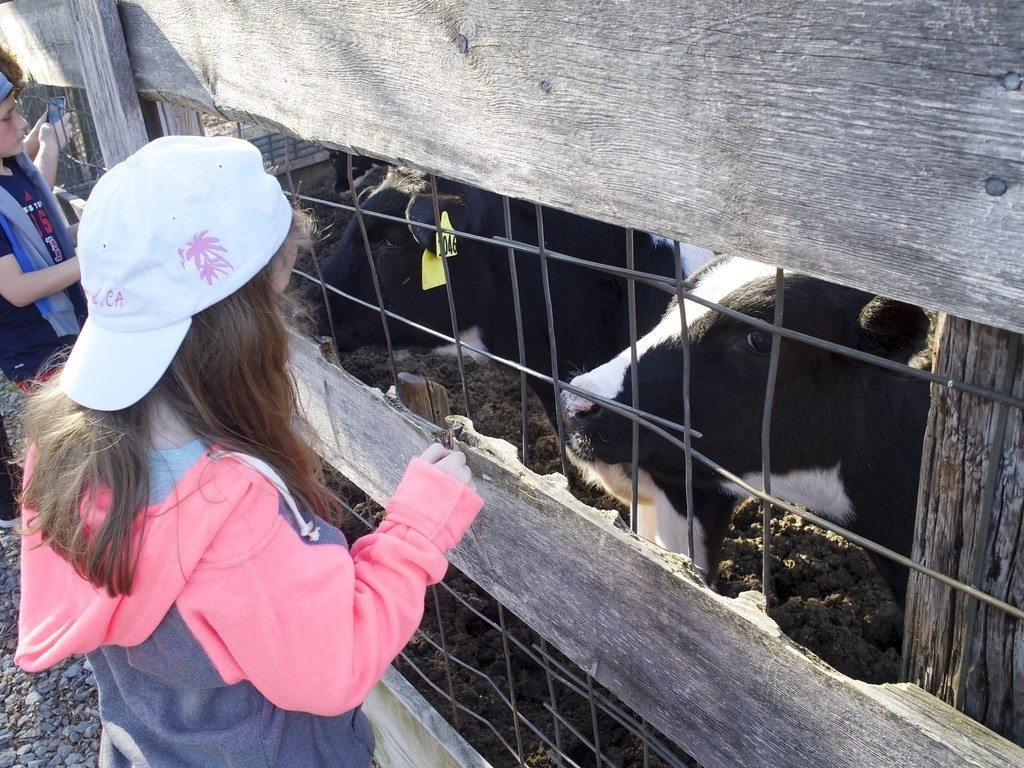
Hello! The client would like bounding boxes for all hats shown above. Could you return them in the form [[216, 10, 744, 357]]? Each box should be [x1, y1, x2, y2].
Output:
[[59, 135, 293, 412], [0, 72, 13, 103]]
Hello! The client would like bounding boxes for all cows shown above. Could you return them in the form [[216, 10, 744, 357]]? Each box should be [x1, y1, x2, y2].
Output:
[[290, 146, 932, 619]]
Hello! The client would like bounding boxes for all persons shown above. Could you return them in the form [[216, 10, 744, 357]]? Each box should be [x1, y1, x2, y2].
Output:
[[13, 135, 483, 768], [0, 51, 89, 530]]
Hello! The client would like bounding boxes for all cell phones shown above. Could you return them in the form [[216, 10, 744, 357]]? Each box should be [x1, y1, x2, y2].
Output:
[[47, 96, 66, 124]]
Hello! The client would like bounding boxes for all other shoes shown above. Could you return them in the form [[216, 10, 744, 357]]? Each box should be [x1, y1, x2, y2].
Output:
[[0, 504, 22, 528]]
